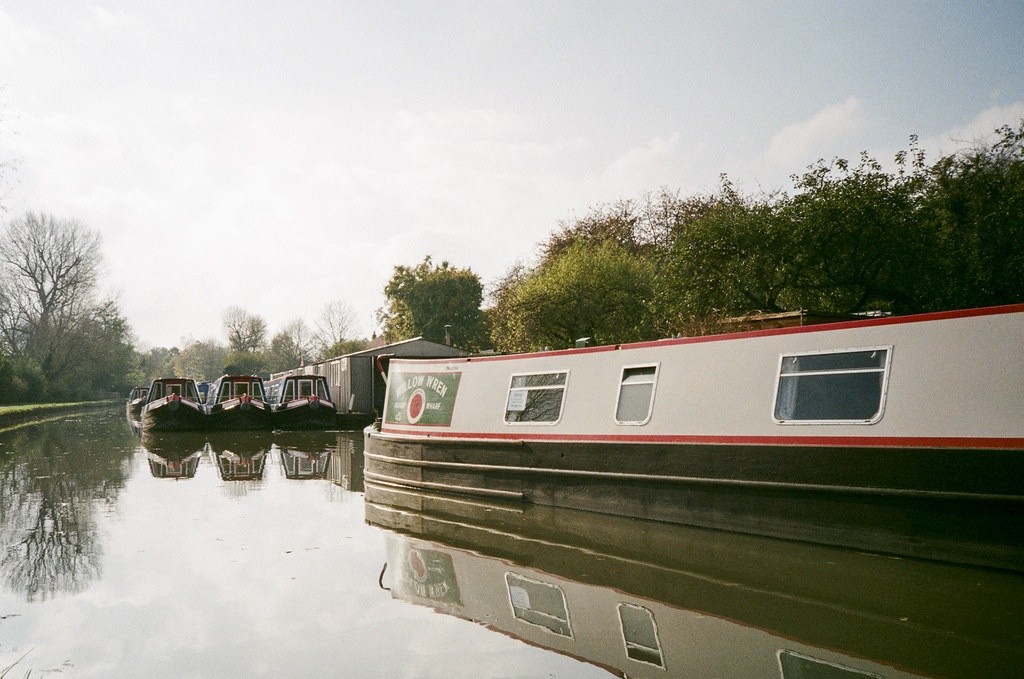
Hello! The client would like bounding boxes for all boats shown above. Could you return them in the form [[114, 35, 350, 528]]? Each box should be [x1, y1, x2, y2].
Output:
[[139, 376, 207, 431], [360, 302, 1024, 570], [272, 430, 338, 481], [361, 478, 1022, 679], [140, 431, 207, 480], [263, 374, 339, 430], [127, 387, 150, 414], [207, 374, 271, 430], [209, 431, 275, 481]]
